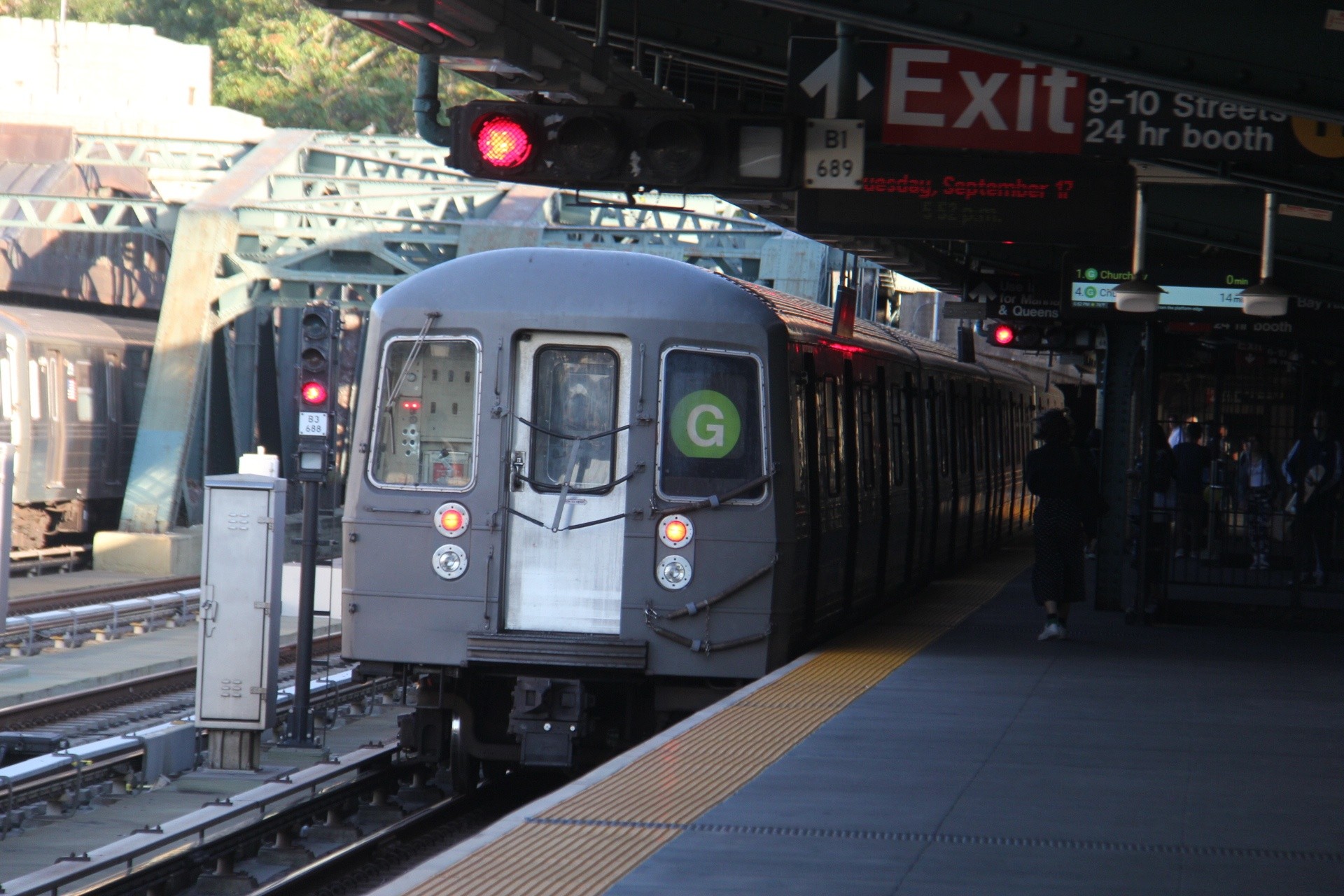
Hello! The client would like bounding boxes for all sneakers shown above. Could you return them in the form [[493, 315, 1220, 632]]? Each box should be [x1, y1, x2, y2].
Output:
[[1037, 621, 1070, 641]]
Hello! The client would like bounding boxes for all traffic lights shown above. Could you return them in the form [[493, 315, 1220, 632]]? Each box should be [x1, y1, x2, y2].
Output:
[[296, 304, 340, 479], [985, 322, 1062, 352], [448, 103, 799, 194]]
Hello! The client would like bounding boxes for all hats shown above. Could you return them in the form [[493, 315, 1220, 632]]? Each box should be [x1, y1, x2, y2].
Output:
[[1034, 410, 1073, 441]]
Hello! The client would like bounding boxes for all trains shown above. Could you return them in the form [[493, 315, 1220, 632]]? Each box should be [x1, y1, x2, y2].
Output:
[[334, 250, 1087, 783]]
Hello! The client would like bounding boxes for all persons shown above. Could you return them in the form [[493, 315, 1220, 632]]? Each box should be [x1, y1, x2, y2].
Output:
[[1025, 409, 1086, 640], [1124, 405, 1342, 622]]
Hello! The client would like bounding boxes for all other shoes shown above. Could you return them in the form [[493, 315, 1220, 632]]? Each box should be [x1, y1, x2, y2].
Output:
[[1249, 559, 1270, 570]]
